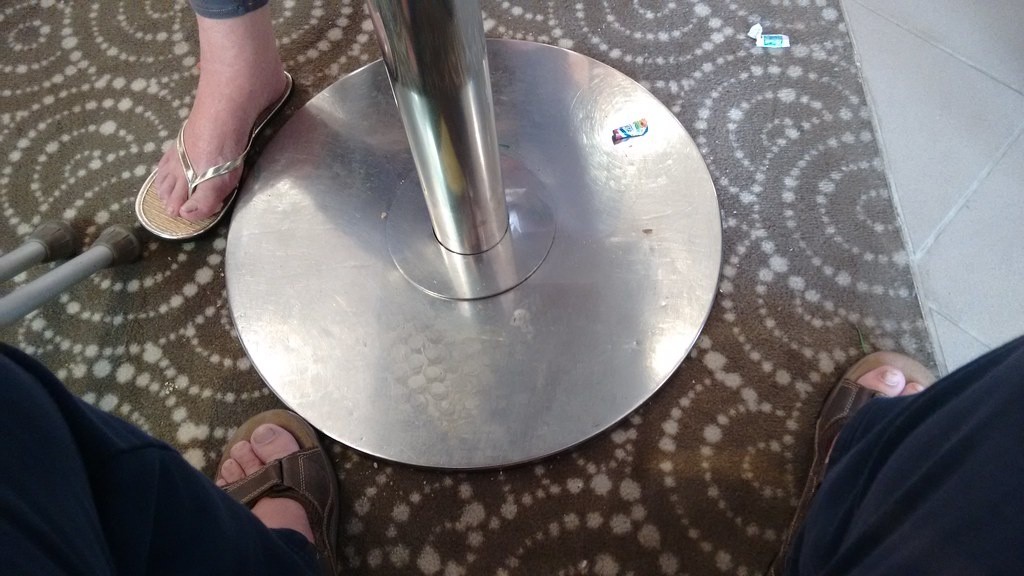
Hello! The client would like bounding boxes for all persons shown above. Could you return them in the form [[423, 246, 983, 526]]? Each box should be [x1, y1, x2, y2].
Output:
[[1, 338, 1023, 575], [133, 0, 294, 240]]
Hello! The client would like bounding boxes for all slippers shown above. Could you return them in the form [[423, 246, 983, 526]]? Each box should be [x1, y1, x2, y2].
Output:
[[134, 68, 294, 240]]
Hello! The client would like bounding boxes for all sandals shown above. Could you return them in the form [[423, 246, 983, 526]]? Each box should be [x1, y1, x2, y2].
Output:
[[210, 410, 338, 576], [776, 352, 939, 575]]
